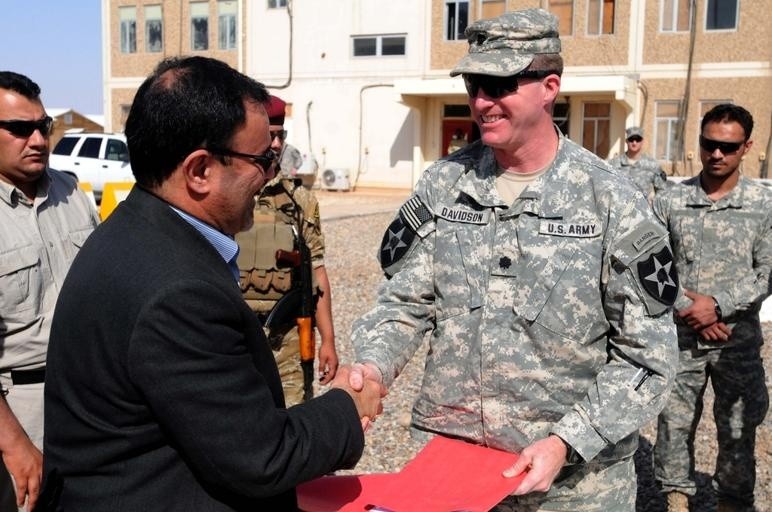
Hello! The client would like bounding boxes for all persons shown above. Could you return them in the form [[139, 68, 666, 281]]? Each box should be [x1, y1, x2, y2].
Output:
[[0, 71, 103, 512], [32, 57, 387, 511], [650, 103, 772, 512], [232, 96, 338, 408], [609, 127, 666, 201], [0, 388, 43, 512], [347, 7, 680, 512]]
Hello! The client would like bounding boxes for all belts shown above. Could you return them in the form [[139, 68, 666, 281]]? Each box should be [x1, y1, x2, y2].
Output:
[[10, 367, 46, 385]]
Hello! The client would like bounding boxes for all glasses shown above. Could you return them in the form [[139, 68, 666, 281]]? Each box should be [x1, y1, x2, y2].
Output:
[[270, 130, 287, 140], [208, 148, 275, 171], [465, 73, 549, 97], [628, 137, 643, 142], [699, 135, 747, 152], [0, 116, 53, 137]]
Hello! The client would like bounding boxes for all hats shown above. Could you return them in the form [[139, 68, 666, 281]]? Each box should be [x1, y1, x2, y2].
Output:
[[264, 95, 286, 117], [626, 127, 644, 138], [450, 8, 562, 76]]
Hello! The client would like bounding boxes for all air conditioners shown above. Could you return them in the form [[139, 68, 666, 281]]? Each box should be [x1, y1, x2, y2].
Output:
[[319, 166, 350, 190]]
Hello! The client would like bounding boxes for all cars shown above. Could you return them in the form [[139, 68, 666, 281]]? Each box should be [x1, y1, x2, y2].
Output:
[[48, 132, 142, 202]]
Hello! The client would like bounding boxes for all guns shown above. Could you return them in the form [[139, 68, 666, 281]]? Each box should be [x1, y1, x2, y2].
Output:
[[262, 174, 316, 402]]
